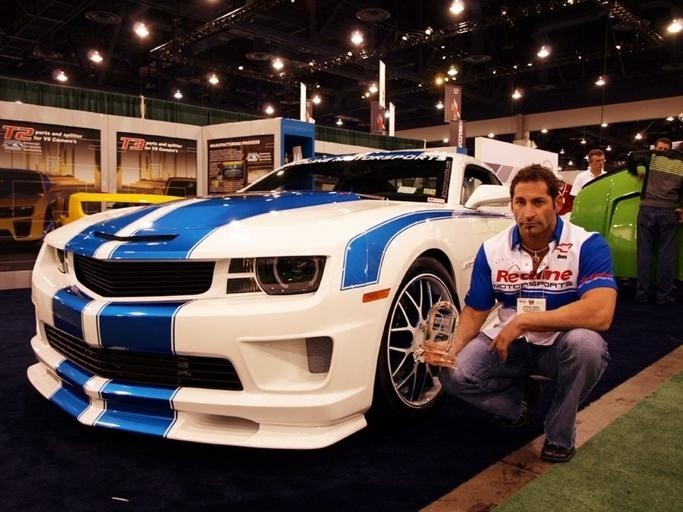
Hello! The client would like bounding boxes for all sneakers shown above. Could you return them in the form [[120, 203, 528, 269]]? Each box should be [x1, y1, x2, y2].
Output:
[[635, 294, 649, 303], [655, 295, 677, 305], [622, 279, 634, 286]]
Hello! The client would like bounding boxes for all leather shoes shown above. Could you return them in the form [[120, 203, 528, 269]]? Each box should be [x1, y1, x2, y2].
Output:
[[540, 437, 576, 462], [501, 378, 543, 428]]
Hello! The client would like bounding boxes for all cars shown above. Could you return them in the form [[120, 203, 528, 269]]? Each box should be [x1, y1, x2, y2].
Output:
[[570, 163, 683, 284], [26, 151, 515, 450], [1, 169, 65, 243]]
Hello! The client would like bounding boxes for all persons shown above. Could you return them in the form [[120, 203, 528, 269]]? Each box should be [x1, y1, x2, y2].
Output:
[[625, 141, 683, 306], [568, 149, 608, 198], [433, 162, 618, 462], [655, 137, 672, 151]]
